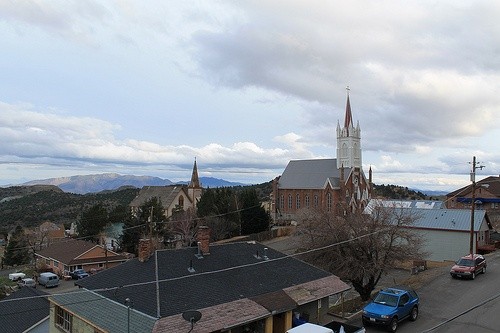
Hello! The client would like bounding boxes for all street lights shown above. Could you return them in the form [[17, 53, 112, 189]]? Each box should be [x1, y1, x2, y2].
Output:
[[469, 183, 491, 255]]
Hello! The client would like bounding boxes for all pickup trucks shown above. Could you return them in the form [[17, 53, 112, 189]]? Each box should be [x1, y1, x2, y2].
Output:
[[284, 320, 367, 333]]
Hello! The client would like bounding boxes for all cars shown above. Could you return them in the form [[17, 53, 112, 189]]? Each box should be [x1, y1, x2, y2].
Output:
[[69, 269, 90, 280], [89, 267, 103, 275]]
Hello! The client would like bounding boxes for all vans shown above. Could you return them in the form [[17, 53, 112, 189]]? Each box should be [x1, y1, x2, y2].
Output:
[[16, 278, 37, 289], [37, 272, 59, 289]]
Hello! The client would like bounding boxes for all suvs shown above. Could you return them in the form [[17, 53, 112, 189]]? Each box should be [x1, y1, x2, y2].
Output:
[[361, 285, 420, 333], [450, 253, 487, 280]]
